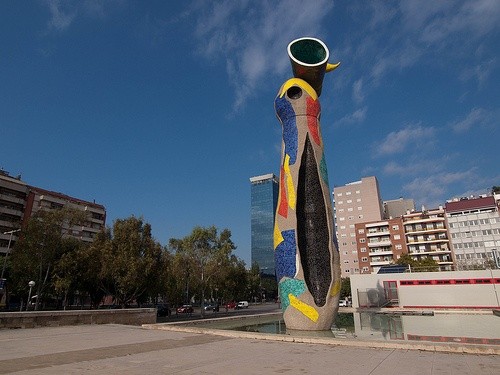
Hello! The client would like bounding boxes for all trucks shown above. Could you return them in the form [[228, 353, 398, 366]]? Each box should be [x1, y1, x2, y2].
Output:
[[238, 301, 248, 310]]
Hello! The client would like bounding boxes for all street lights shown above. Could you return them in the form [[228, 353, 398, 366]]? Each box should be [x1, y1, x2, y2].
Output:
[[26, 279, 36, 311], [1, 229, 22, 279]]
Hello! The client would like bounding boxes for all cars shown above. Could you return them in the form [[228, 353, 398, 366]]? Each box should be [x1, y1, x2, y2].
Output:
[[177, 304, 194, 313], [226, 303, 238, 310], [205, 303, 219, 311], [339, 299, 348, 307]]
